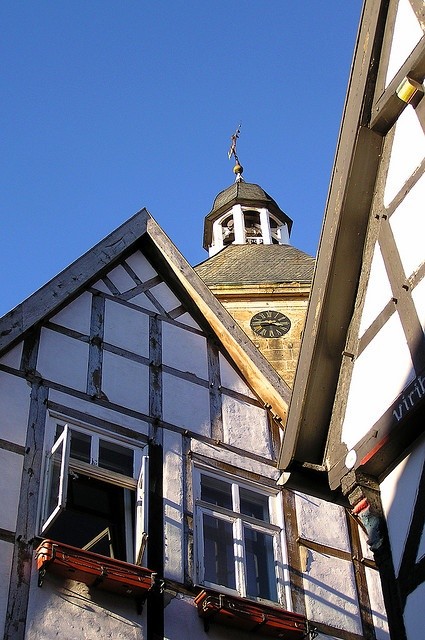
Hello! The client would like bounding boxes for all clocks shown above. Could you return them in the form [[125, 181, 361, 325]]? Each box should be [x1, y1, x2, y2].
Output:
[[250, 310, 291, 338]]
